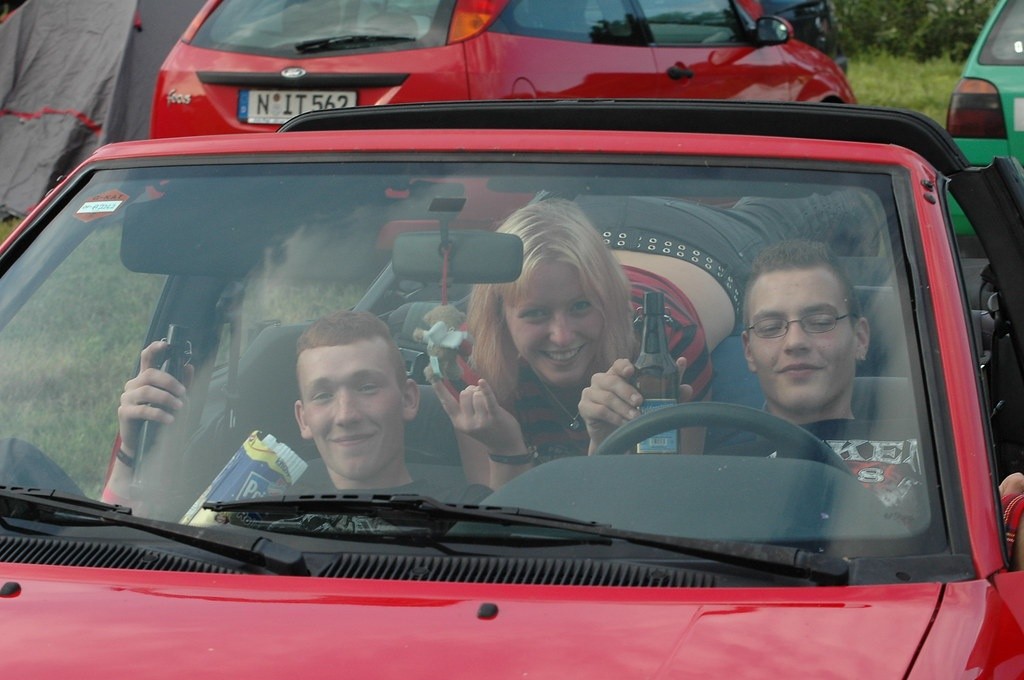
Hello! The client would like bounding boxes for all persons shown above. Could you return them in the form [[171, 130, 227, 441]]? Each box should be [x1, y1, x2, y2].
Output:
[[97, 309, 494, 528], [575, 237, 1024, 574], [425, 180, 883, 490]]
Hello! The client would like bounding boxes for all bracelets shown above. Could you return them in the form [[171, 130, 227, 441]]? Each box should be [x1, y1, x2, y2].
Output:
[[117, 450, 136, 468], [103, 485, 134, 508], [489, 444, 539, 466]]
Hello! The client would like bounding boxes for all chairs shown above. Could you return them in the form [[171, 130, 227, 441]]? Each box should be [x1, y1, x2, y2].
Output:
[[711, 258, 999, 443], [235, 301, 463, 504]]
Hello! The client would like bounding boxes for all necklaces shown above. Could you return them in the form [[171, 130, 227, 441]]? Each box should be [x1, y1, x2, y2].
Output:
[[541, 383, 581, 431]]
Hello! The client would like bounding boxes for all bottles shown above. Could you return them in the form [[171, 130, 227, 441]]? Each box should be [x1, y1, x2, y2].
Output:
[[133, 324, 187, 517], [631, 292, 681, 455]]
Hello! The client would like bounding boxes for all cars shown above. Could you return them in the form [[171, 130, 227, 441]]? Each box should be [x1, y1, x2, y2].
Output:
[[0, 97, 1024, 680], [148, 0, 863, 202], [931, 0, 1024, 259]]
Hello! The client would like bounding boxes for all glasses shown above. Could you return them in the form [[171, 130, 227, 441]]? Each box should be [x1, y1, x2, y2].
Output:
[[747, 312, 852, 340]]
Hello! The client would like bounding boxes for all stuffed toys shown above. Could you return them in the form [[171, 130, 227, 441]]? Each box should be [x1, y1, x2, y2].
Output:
[[411, 305, 478, 382]]
